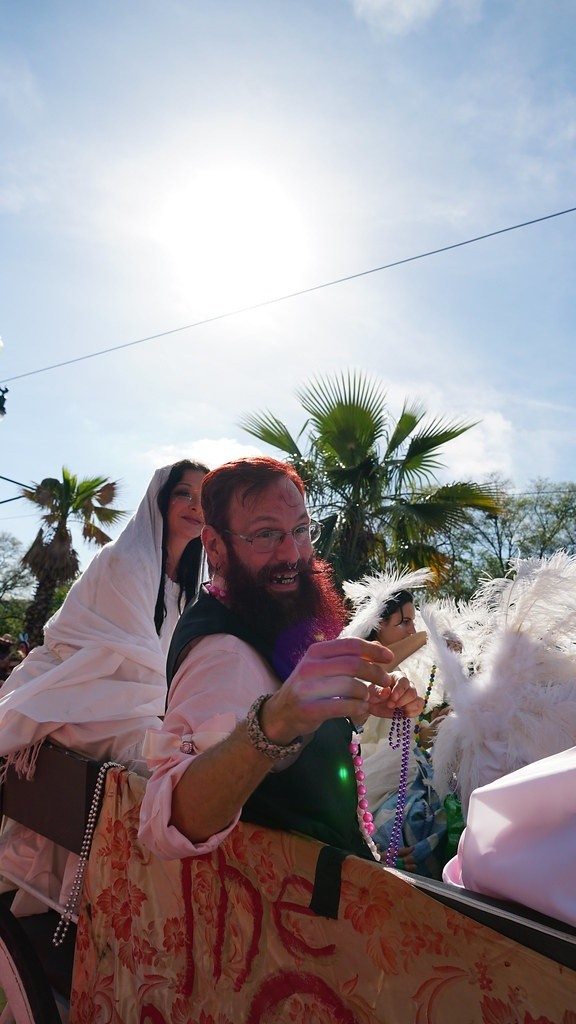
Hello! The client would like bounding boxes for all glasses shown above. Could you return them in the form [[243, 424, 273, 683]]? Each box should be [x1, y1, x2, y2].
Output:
[[165, 495, 201, 504], [224, 518, 326, 554]]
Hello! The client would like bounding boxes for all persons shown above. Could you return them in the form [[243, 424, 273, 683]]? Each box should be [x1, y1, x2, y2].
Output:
[[341, 567, 463, 876], [138, 456, 425, 872], [0, 457, 212, 920], [0, 633, 40, 685]]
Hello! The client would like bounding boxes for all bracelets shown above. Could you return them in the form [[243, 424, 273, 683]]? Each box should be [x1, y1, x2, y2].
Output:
[[246, 693, 301, 759]]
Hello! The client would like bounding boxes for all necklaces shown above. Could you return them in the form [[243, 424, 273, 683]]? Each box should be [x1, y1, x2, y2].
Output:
[[414, 662, 437, 743], [53, 762, 124, 945], [386, 708, 412, 867], [351, 731, 383, 863]]
